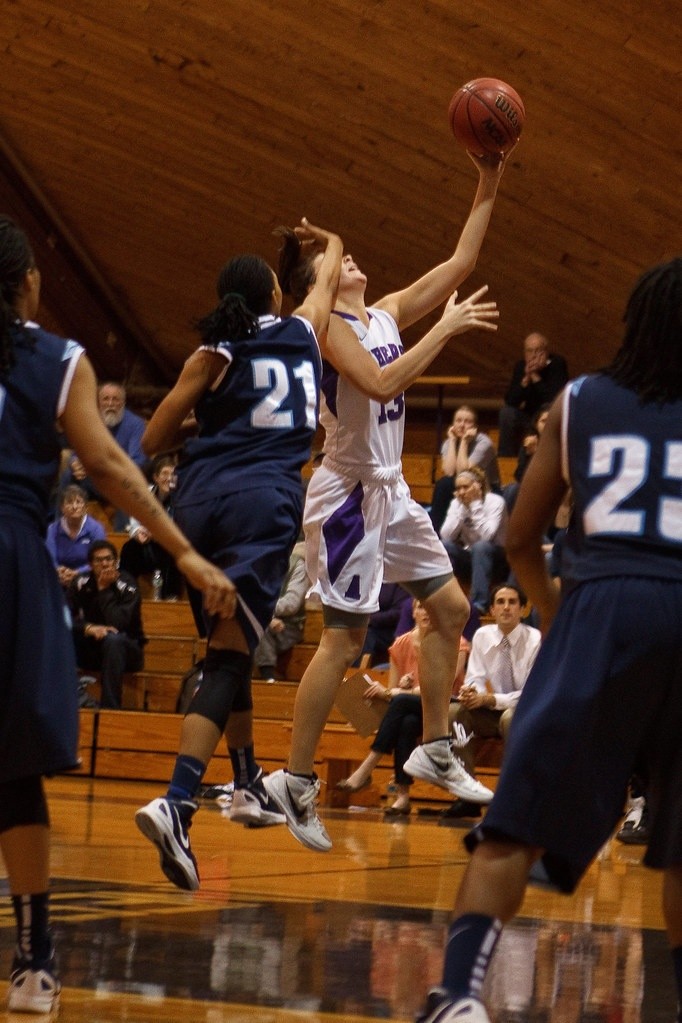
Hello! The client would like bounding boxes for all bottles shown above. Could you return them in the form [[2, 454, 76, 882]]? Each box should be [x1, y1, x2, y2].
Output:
[[387, 774, 400, 807], [151, 569, 164, 601]]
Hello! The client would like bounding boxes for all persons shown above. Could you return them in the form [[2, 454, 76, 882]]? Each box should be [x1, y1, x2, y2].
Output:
[[615, 785, 651, 843], [439, 469, 507, 616], [138, 216, 345, 891], [429, 333, 572, 575], [261, 138, 521, 852], [254, 529, 311, 683], [50, 381, 200, 706], [340, 585, 543, 817], [0, 225, 235, 1013], [414, 258, 682, 1023]]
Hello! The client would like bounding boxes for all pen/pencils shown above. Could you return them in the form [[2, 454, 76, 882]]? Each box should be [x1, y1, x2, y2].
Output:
[[458, 680, 473, 699], [364, 673, 372, 684]]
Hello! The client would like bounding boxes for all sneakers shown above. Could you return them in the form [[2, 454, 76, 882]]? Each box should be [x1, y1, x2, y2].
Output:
[[262, 769, 333, 852], [403, 738, 495, 804], [616, 795, 646, 843], [229, 766, 288, 826], [134, 796, 200, 891]]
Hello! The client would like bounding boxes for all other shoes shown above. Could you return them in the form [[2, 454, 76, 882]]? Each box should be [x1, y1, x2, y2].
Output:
[[385, 802, 410, 815], [7, 953, 62, 1013], [415, 985, 489, 1023], [337, 774, 372, 793], [439, 799, 481, 818]]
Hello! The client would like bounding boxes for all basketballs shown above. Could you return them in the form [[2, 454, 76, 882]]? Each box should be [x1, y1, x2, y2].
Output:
[[447, 77, 526, 154]]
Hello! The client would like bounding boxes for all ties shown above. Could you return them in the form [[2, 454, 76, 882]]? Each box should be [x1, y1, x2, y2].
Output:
[[500, 637, 514, 694]]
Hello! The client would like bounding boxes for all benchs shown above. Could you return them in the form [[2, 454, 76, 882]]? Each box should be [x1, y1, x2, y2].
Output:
[[68, 456, 634, 831]]
